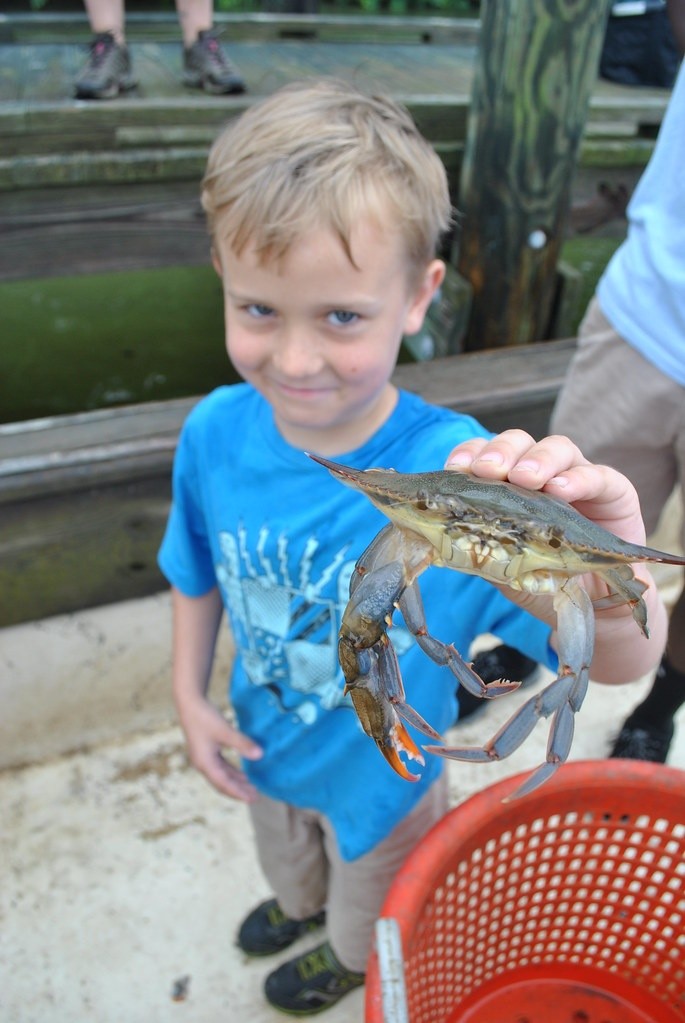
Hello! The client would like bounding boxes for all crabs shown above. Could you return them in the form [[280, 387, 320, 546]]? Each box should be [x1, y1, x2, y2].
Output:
[[303, 450, 685, 805]]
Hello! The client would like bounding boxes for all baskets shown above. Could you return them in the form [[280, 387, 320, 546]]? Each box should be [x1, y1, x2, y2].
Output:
[[362, 756, 685, 1023]]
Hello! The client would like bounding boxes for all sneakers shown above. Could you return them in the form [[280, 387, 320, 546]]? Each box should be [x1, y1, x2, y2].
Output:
[[458, 647, 543, 724], [261, 940, 368, 1017], [76, 32, 140, 101], [182, 30, 247, 94], [236, 898, 327, 960], [607, 704, 674, 763]]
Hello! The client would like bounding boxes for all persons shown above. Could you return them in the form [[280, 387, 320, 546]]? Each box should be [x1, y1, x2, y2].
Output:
[[432, 46, 685, 771], [73, 0, 252, 100], [149, 77, 670, 1018]]
